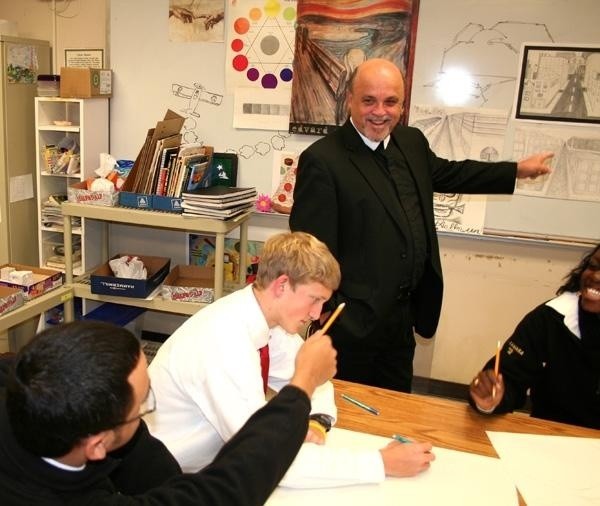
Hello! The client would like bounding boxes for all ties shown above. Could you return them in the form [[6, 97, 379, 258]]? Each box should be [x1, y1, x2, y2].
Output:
[[259, 344, 270, 390]]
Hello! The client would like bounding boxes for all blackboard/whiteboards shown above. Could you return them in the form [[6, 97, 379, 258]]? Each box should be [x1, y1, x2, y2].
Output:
[[108, 0, 600, 250]]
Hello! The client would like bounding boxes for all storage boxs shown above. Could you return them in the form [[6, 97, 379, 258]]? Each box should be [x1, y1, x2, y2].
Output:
[[59, 66, 114, 99]]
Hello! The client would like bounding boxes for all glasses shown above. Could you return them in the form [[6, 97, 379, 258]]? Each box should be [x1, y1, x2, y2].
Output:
[[103, 383, 156, 432]]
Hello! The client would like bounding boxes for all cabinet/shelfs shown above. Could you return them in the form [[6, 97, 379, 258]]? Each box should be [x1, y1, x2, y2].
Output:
[[34, 96, 108, 317], [60, 198, 250, 326]]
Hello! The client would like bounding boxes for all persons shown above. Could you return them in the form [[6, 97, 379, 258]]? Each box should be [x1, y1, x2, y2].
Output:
[[169, 5, 196, 25], [287, 59, 554, 394], [137, 231, 436, 490], [466, 245, 600, 431], [0, 320, 336, 505], [196, 9, 225, 31]]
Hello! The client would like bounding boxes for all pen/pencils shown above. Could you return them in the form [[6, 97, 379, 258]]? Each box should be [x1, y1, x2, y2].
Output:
[[392, 435, 411, 443], [340, 394, 379, 415], [492, 340, 502, 399], [321, 303, 345, 334]]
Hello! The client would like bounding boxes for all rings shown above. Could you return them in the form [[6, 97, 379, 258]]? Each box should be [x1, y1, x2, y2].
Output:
[[475, 378, 479, 388]]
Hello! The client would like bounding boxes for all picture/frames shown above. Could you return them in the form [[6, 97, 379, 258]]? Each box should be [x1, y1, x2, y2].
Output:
[[515, 45, 600, 123]]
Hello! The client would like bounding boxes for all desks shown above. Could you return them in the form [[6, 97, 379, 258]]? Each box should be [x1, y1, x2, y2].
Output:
[[264, 374, 600, 506], [0, 286, 75, 353]]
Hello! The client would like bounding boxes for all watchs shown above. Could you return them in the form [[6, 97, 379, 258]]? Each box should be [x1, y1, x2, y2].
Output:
[[310, 414, 332, 434]]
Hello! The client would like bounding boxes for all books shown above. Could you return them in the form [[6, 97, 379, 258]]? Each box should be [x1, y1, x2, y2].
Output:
[[124, 109, 258, 223], [42, 144, 81, 269]]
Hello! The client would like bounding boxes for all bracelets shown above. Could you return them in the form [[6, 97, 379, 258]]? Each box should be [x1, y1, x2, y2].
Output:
[[308, 421, 327, 441]]
